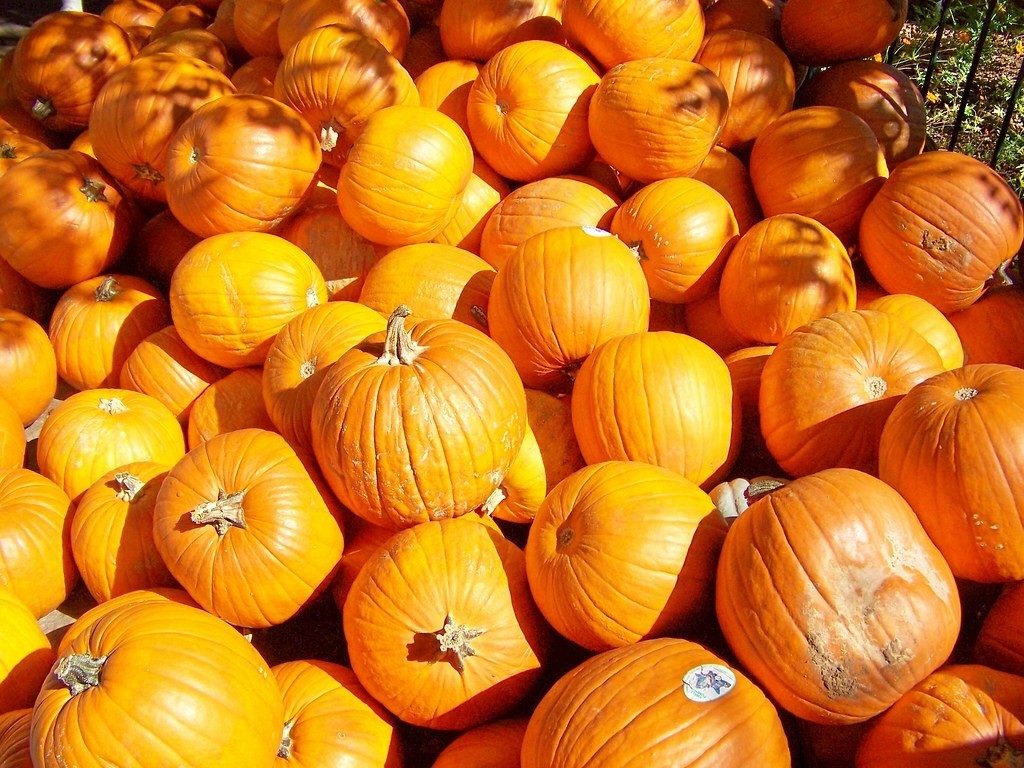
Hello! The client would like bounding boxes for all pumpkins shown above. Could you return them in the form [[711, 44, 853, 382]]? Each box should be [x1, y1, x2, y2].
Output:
[[0, 0, 1024, 768]]
